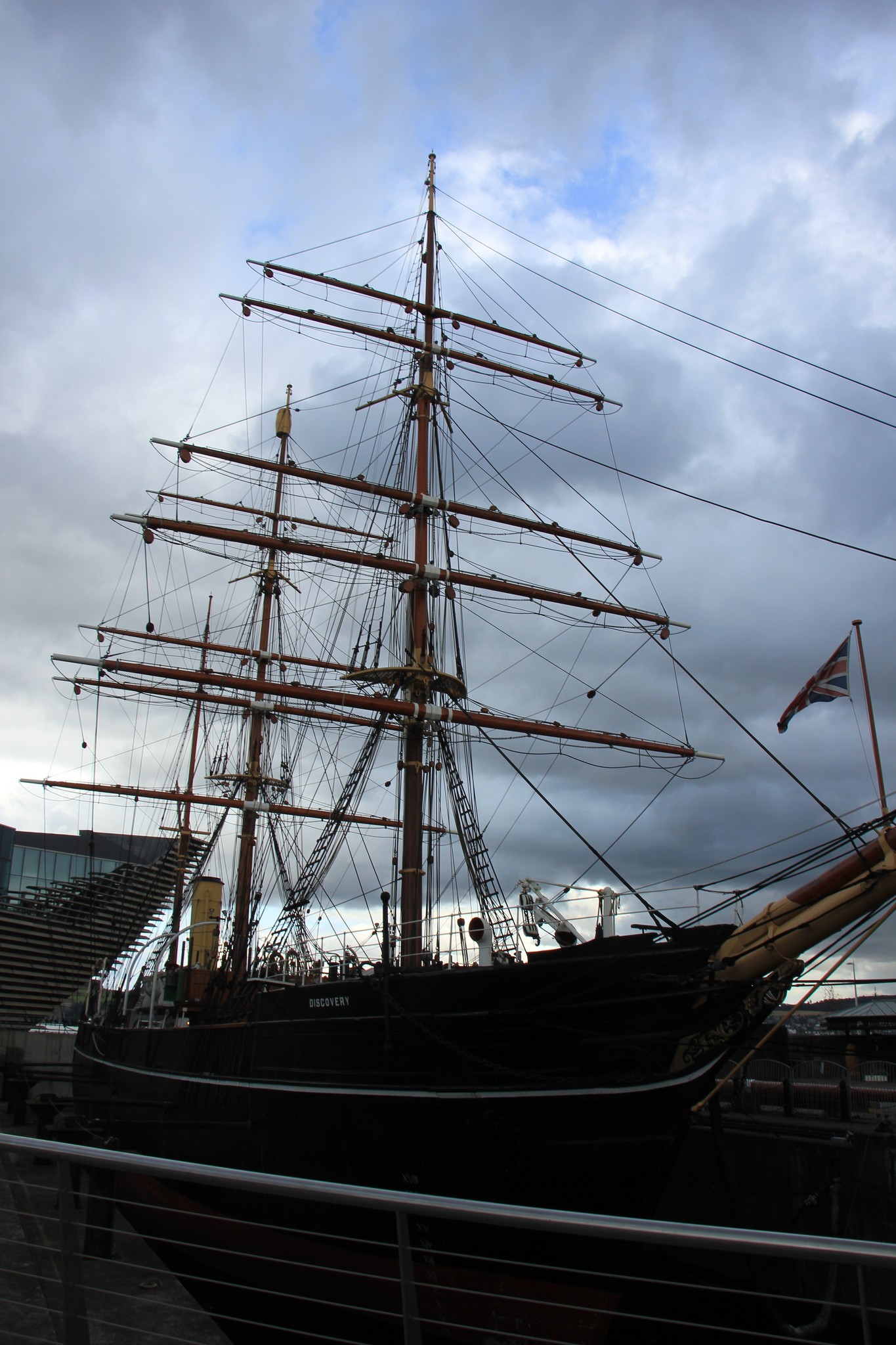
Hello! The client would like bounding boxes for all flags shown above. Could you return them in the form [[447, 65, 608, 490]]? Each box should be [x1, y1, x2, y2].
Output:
[[777, 630, 853, 734]]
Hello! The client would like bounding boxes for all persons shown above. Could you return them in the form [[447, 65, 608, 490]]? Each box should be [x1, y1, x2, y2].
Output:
[[846, 1030, 851, 1040], [87, 1018, 92, 1024], [121, 1023, 125, 1028]]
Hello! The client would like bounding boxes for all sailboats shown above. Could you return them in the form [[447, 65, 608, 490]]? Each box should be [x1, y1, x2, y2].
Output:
[[17, 153, 896, 1344]]
